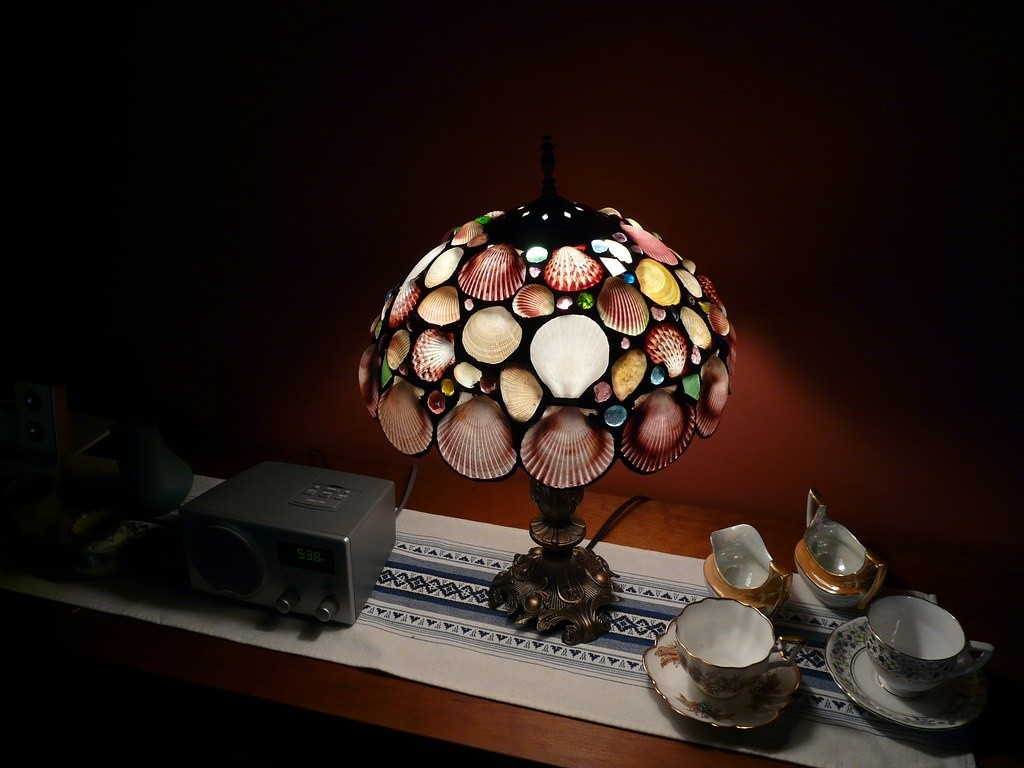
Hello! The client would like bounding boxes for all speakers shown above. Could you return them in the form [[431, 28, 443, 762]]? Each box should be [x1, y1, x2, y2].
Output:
[[14, 382, 71, 454]]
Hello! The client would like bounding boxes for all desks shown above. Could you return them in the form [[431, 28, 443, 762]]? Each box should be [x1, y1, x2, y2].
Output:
[[0, 471, 1024, 768]]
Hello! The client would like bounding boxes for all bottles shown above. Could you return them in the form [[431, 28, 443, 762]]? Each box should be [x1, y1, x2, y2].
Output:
[[5, 473, 135, 576]]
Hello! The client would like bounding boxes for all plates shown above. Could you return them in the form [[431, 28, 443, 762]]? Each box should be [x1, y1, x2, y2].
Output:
[[643, 618, 800, 729], [824, 616, 990, 731]]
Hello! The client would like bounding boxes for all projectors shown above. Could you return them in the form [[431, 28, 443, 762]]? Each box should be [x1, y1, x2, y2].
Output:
[[176, 458, 395, 626]]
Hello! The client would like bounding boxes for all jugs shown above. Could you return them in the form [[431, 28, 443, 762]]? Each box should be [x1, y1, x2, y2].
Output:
[[702, 523, 794, 621]]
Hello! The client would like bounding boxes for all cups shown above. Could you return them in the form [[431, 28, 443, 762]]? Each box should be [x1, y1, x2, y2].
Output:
[[864, 593, 995, 701], [793, 488, 889, 610], [676, 597, 809, 699]]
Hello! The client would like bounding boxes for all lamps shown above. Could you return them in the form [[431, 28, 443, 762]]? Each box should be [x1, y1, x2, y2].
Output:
[[356, 130, 741, 646]]
